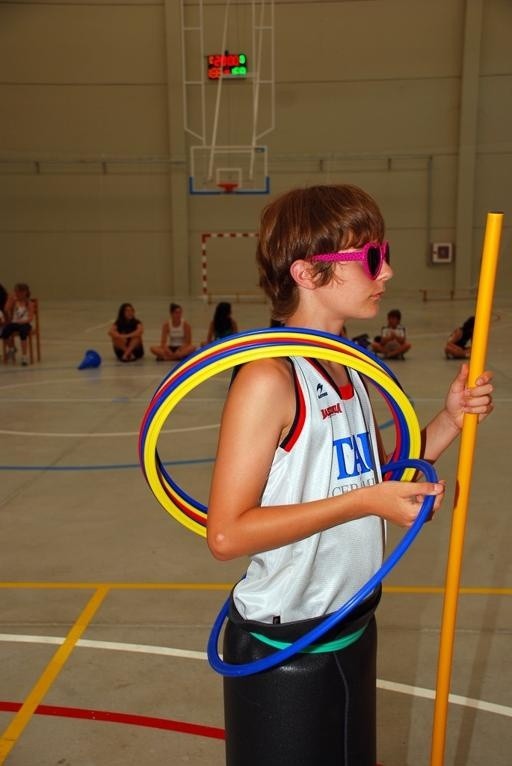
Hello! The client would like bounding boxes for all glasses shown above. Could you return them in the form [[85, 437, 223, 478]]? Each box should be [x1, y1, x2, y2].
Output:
[[311, 240, 390, 280]]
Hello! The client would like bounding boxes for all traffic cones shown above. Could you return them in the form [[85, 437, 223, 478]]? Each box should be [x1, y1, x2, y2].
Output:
[[78, 350, 100, 369]]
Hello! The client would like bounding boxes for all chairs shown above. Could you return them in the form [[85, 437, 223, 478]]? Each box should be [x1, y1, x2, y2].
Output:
[[3, 298, 41, 364]]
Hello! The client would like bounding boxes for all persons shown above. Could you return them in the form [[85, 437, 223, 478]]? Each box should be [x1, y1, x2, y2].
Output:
[[444, 316, 475, 359], [206, 183, 495, 766], [372, 309, 411, 359], [150, 302, 196, 360], [0, 282, 35, 366], [107, 302, 144, 362], [200, 302, 238, 346]]
